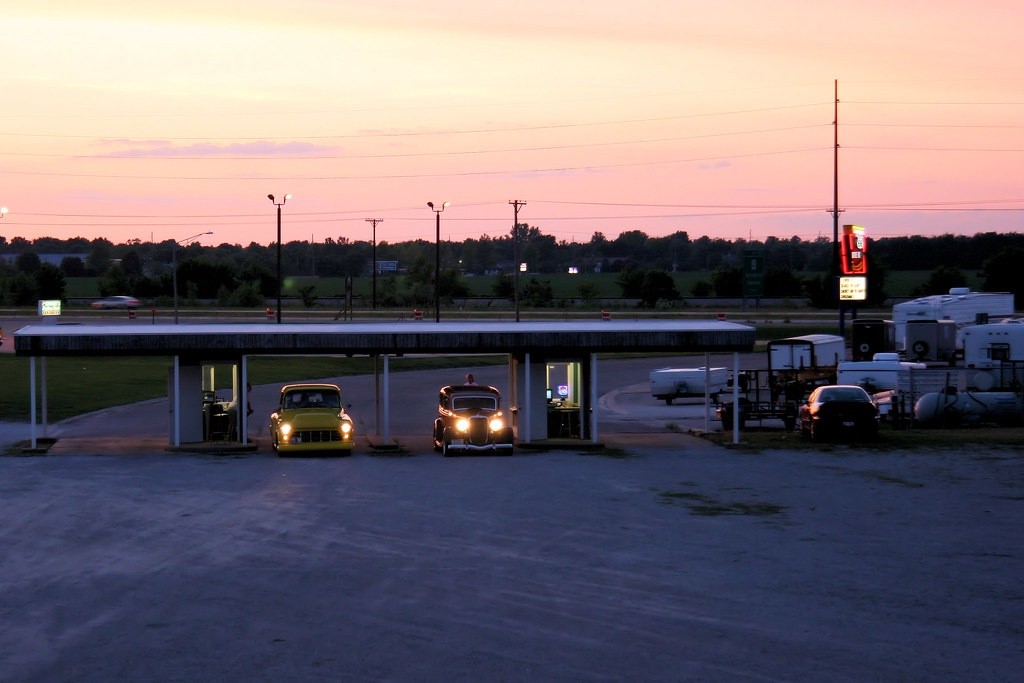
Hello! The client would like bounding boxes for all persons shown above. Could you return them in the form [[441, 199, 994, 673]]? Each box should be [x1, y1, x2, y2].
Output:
[[227, 382, 253, 443], [464, 373, 478, 386], [797, 401, 814, 443]]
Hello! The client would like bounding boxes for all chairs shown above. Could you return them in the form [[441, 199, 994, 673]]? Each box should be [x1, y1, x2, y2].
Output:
[[318, 401, 331, 408]]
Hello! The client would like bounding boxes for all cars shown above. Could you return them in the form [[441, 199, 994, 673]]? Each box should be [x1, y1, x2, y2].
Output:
[[267, 384, 354, 453], [797, 385, 881, 440], [434, 383, 515, 453], [90, 295, 143, 310]]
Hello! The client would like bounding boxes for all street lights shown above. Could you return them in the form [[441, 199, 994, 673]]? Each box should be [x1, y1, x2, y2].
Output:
[[426, 201, 452, 323], [173, 230, 215, 326], [266, 194, 293, 325]]
